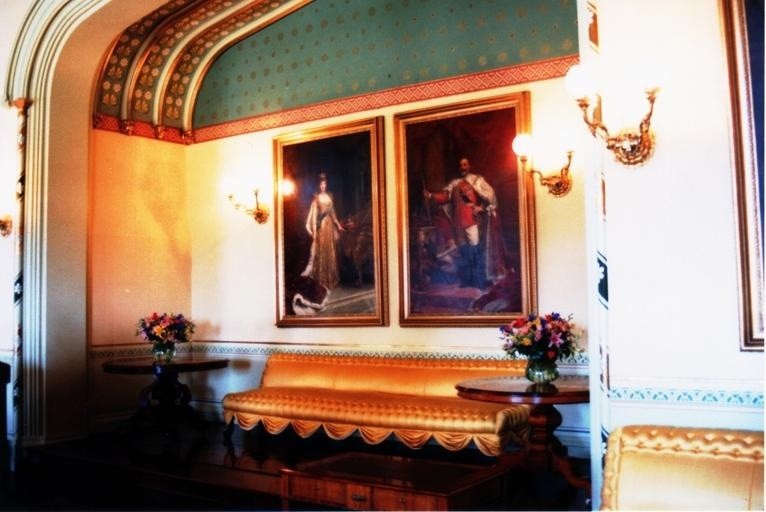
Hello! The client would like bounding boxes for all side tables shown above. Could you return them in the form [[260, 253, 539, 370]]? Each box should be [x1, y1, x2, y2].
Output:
[[456, 374, 589, 499], [103, 356, 230, 471]]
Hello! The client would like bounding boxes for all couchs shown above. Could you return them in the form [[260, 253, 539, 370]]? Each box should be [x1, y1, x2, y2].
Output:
[[219, 352, 538, 470]]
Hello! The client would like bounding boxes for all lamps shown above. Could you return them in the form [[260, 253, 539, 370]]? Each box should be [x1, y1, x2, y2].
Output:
[[225, 176, 270, 225], [563, 50, 671, 164], [511, 118, 578, 196]]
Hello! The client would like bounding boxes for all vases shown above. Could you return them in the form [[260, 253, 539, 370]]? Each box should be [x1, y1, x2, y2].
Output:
[[526, 353, 559, 396], [154, 344, 173, 363]]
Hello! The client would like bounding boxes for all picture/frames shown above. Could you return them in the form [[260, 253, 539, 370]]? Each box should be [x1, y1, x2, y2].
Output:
[[393, 91, 537, 328], [719, 1, 765, 352], [271, 116, 390, 327]]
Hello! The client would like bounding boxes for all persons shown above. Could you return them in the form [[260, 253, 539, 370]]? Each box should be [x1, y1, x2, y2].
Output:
[[300, 171, 346, 288], [422, 156, 498, 291]]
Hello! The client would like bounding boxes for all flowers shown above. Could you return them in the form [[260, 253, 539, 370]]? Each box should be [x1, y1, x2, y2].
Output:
[[498, 313, 587, 364], [134, 310, 195, 353]]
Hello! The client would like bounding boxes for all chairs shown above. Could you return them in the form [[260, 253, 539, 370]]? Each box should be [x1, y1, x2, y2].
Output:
[[598, 425, 764, 511]]
